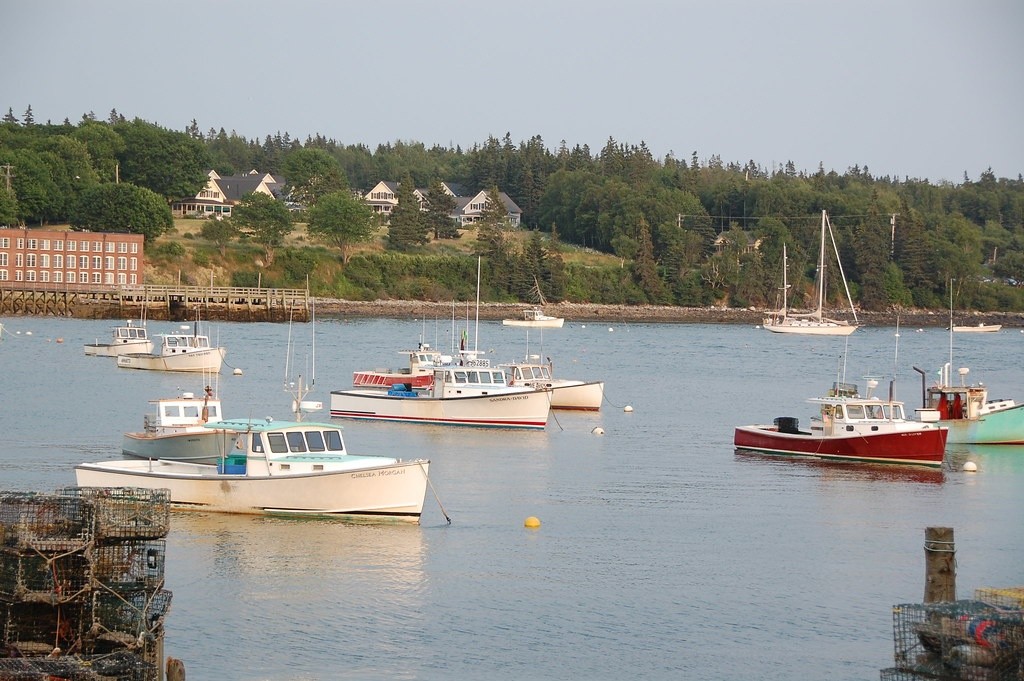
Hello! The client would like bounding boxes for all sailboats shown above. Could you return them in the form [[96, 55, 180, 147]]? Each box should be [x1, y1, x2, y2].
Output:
[[761, 209, 865, 336]]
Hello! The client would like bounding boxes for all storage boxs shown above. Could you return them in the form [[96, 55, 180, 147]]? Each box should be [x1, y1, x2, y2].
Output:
[[880, 586, 1023, 681], [0, 484, 172, 680]]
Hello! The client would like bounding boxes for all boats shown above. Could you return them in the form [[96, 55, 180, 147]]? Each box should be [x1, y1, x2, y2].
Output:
[[115, 297, 226, 374], [328, 254, 555, 427], [910, 278, 1024, 449], [499, 307, 566, 328], [493, 322, 605, 412], [71, 273, 433, 524], [732, 330, 950, 469], [952, 323, 1003, 332], [78, 316, 155, 357]]
